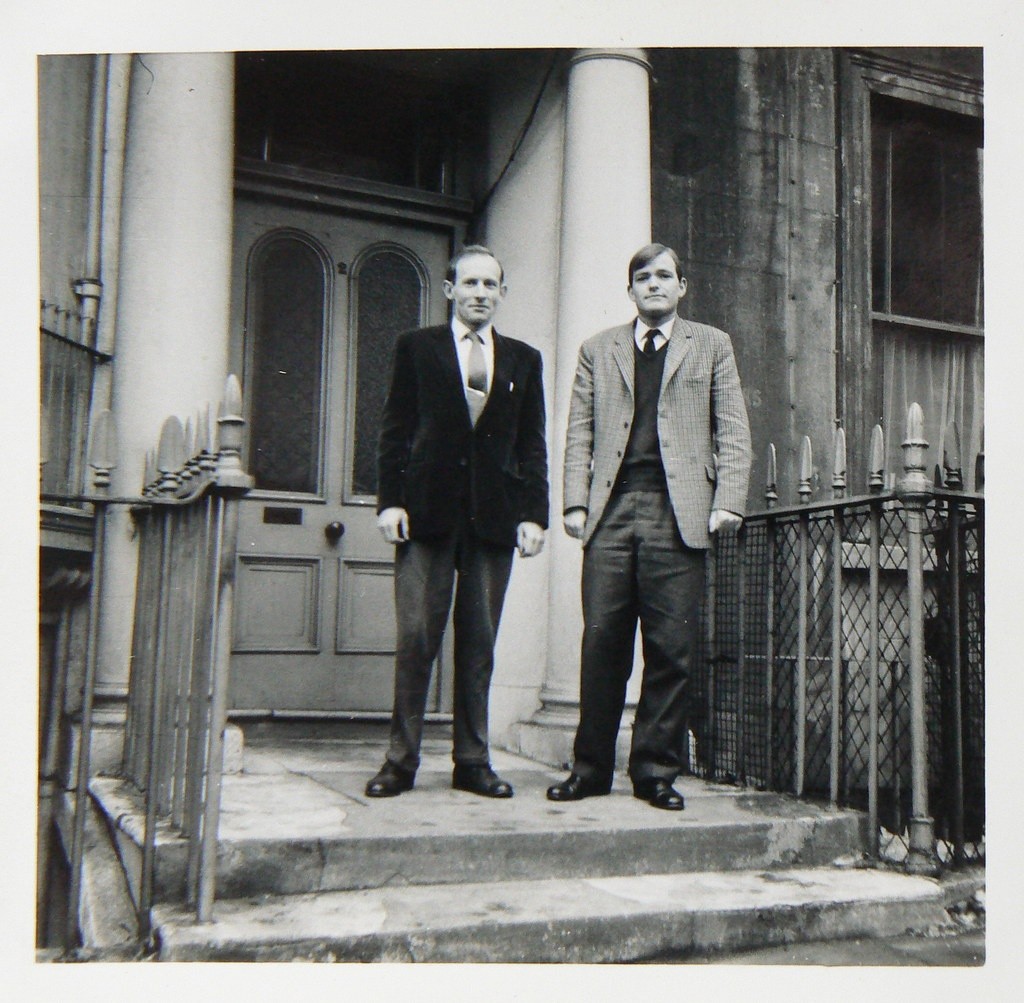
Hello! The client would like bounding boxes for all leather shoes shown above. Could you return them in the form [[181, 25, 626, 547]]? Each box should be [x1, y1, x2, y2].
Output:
[[632, 774, 684, 810], [365, 759, 416, 798], [547, 771, 613, 801], [452, 761, 513, 798]]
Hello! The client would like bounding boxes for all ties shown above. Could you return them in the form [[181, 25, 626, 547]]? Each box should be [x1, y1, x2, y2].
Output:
[[643, 329, 661, 356], [464, 331, 488, 394]]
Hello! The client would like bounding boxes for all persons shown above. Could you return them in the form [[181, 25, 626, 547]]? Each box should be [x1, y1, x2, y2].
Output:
[[364, 242, 549, 799], [548, 244, 753, 811]]
